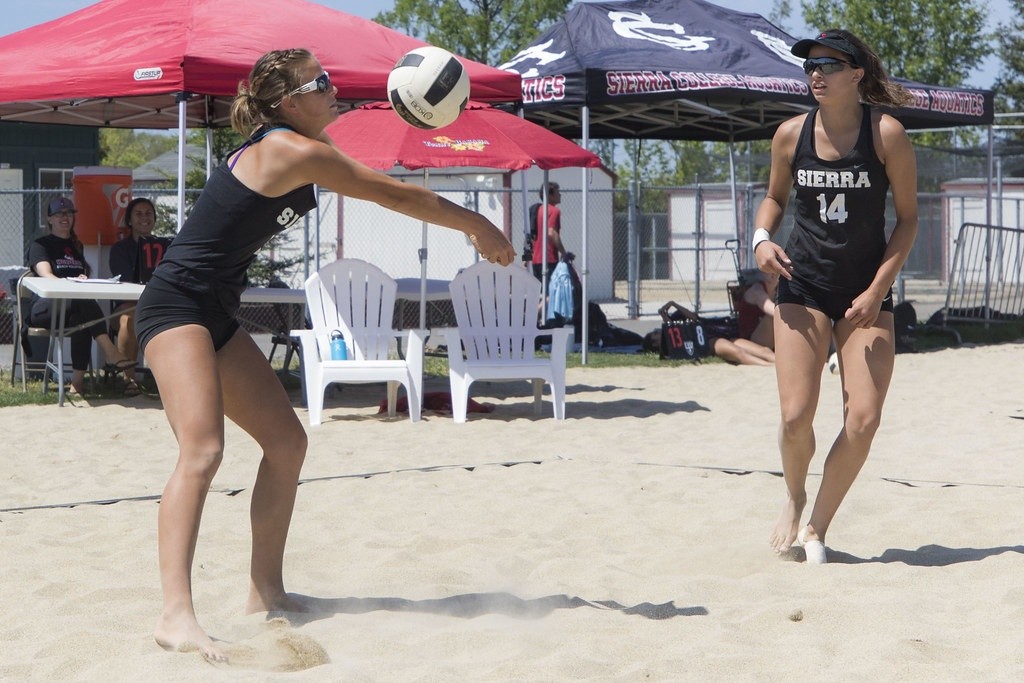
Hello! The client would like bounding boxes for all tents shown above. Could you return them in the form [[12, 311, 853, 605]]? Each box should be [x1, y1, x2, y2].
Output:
[[491, 0, 994, 365], [0, 0, 522, 235]]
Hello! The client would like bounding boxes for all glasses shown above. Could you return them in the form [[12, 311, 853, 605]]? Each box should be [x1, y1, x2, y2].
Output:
[[802, 56, 860, 76], [271, 71, 331, 109], [53, 211, 75, 218]]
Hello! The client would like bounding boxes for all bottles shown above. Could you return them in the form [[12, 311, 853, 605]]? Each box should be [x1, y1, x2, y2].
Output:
[[330, 329, 347, 361]]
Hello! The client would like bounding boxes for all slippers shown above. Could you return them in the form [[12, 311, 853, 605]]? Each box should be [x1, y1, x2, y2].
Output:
[[102, 358, 140, 371]]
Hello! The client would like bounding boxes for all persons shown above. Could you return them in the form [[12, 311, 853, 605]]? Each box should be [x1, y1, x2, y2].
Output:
[[525, 182, 570, 326], [752, 29, 919, 564], [134, 49, 517, 662], [738, 257, 780, 352], [642, 301, 775, 365], [30, 198, 139, 397], [109, 198, 172, 396]]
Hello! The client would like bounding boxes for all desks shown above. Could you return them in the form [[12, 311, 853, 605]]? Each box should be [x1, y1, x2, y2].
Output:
[[20, 277, 452, 407]]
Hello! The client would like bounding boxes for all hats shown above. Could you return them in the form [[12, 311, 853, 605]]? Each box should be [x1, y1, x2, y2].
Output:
[[47, 197, 78, 216], [791, 30, 863, 66]]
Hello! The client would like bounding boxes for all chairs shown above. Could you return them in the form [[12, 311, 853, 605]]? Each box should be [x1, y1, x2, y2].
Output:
[[11, 270, 94, 394], [439, 259, 574, 425], [269, 277, 342, 392], [289, 258, 430, 422], [104, 299, 151, 390]]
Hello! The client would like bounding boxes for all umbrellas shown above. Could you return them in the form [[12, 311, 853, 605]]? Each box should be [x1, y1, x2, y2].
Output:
[[323, 100, 602, 346]]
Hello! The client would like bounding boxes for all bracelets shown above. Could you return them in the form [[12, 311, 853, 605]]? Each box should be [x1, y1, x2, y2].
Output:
[[752, 228, 771, 254]]
